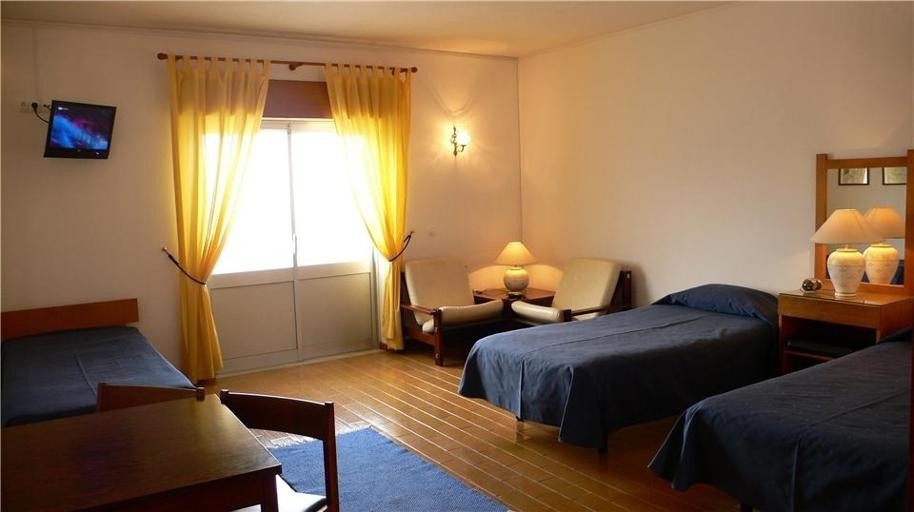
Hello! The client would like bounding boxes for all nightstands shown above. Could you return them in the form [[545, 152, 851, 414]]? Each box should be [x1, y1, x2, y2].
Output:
[[778, 287, 914, 375]]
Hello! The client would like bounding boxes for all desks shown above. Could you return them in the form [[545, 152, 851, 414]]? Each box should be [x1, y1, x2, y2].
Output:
[[1, 394, 282, 512]]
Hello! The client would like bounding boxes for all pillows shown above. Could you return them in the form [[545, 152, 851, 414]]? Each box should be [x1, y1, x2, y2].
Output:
[[651, 284, 778, 323]]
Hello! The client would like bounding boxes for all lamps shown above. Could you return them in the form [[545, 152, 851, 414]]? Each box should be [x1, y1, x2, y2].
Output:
[[810, 208, 881, 296], [494, 241, 534, 299], [863, 207, 905, 285], [450, 126, 469, 156]]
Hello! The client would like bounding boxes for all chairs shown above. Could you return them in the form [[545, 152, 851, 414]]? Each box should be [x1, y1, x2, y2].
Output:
[[510, 257, 632, 323], [400, 257, 507, 367], [220, 389, 339, 512], [96, 383, 205, 412]]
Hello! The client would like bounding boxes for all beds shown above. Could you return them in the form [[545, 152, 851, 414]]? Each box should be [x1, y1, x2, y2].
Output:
[[646, 329, 914, 512], [1, 297, 196, 427], [456, 304, 777, 460]]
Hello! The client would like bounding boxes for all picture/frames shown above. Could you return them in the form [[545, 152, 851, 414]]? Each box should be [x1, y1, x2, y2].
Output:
[[839, 168, 870, 186], [882, 167, 907, 185]]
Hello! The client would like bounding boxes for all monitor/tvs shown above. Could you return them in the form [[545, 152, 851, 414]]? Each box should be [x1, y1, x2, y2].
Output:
[[43, 99, 118, 160]]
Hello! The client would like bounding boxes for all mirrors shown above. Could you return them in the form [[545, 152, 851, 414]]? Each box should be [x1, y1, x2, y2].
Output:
[[815, 149, 914, 297]]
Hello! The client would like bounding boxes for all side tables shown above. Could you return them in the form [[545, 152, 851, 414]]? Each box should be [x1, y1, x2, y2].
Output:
[[474, 288, 555, 331]]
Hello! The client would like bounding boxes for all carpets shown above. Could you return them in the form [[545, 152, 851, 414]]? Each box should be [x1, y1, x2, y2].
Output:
[[268, 426, 511, 512]]
